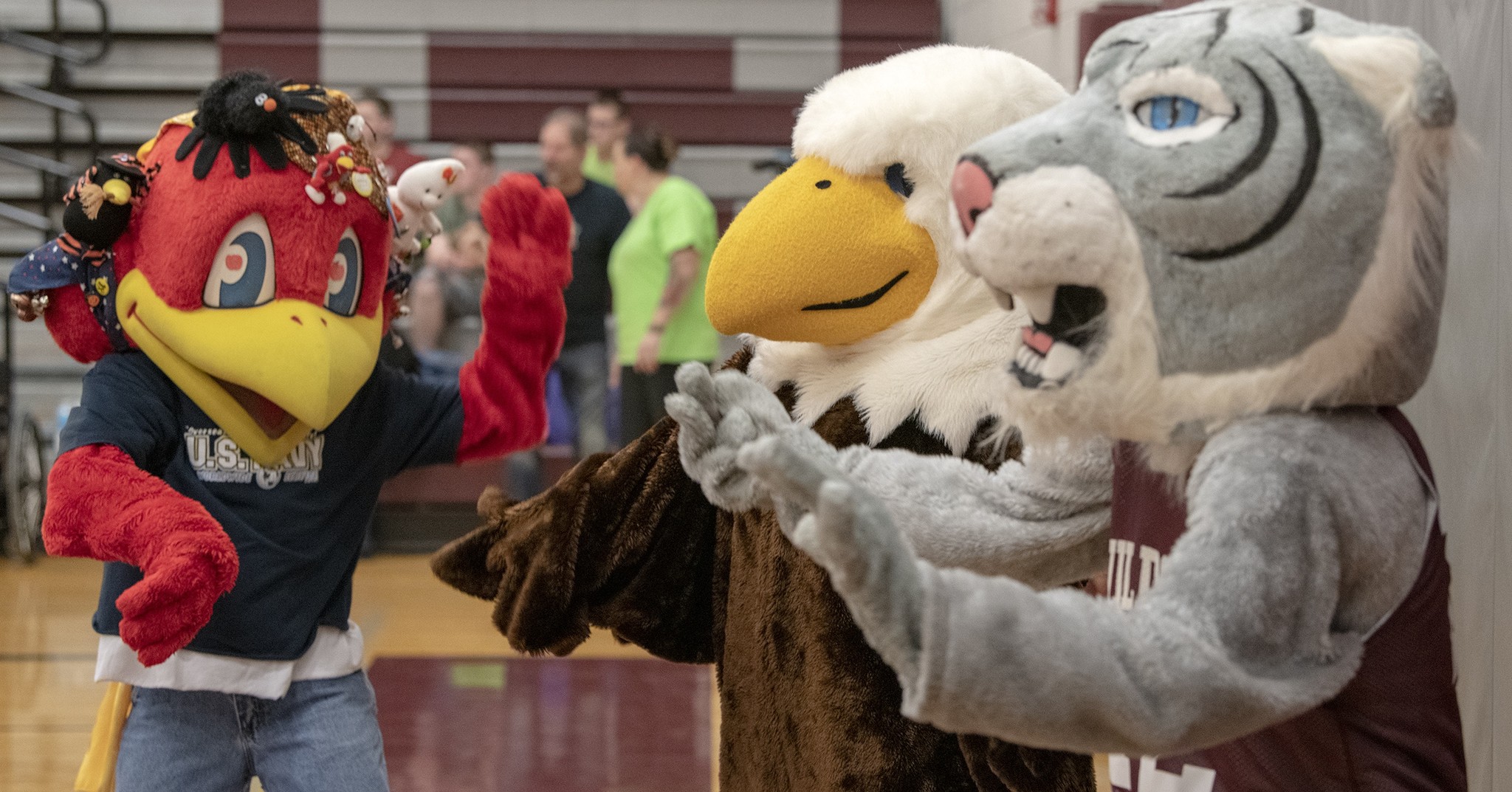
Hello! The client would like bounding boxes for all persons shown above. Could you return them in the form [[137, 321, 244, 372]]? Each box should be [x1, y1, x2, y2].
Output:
[[349, 88, 719, 509]]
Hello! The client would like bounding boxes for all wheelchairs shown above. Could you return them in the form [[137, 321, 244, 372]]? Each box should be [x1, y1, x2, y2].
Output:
[[0, 285, 47, 564]]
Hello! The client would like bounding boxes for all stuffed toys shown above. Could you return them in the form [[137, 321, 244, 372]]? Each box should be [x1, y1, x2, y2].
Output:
[[663, 0, 1471, 791], [426, 45, 1115, 792], [10, 68, 574, 792]]
